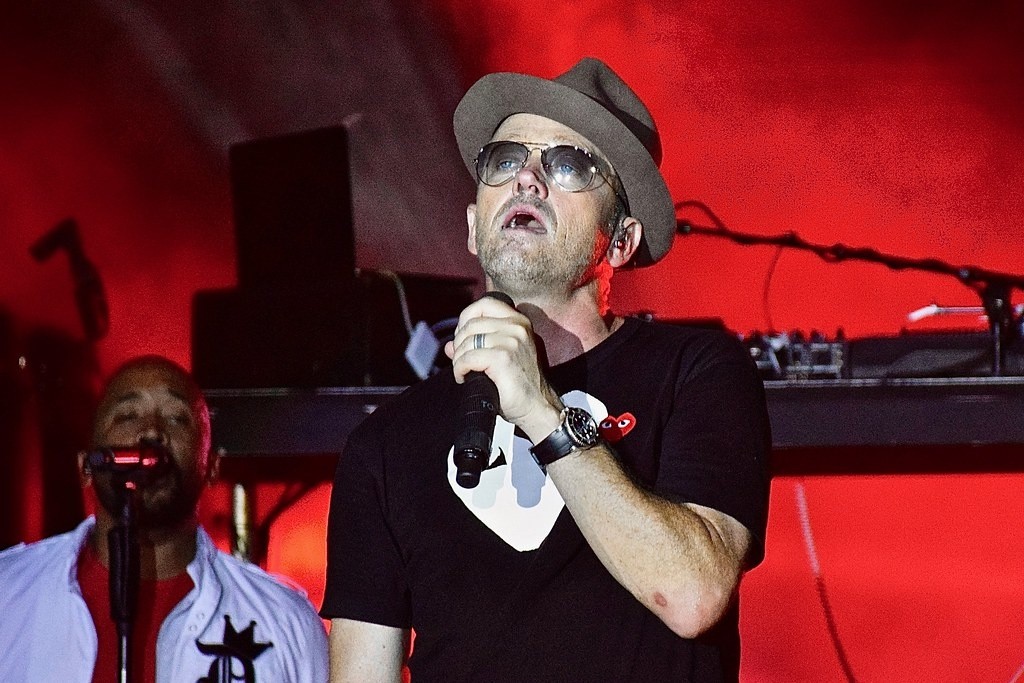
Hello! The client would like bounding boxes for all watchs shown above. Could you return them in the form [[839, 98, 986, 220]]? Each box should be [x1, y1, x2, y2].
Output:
[[527, 407, 600, 474]]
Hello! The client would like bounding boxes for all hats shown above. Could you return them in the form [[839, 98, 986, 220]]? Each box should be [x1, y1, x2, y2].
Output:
[[453, 56, 676, 268]]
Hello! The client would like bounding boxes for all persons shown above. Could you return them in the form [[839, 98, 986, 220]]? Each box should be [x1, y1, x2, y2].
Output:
[[0, 353, 331, 683], [309, 56, 773, 683]]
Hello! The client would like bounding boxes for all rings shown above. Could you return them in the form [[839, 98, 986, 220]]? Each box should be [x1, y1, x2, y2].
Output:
[[472, 332, 486, 350]]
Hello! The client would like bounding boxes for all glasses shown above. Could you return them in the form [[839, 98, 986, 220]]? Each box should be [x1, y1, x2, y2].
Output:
[[473, 141, 630, 218]]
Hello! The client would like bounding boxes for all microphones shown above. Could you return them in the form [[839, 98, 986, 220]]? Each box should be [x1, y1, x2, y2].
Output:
[[86, 442, 171, 474], [451, 291, 514, 488], [675, 219, 690, 235]]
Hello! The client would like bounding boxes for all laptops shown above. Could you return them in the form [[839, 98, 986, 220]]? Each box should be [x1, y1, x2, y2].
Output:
[[226, 126, 479, 287]]
